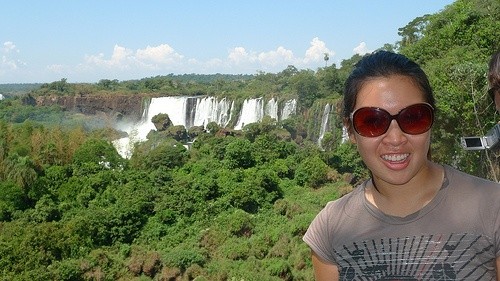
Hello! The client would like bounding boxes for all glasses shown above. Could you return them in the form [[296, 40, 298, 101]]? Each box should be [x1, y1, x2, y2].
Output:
[[487, 85, 499, 100], [349, 101, 435, 137]]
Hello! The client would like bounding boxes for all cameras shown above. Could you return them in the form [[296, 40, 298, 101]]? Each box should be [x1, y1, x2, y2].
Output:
[[461, 124, 500, 151]]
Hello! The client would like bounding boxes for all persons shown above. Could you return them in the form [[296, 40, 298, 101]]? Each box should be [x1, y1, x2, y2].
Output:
[[487, 44, 500, 125], [302, 50, 500, 281]]
